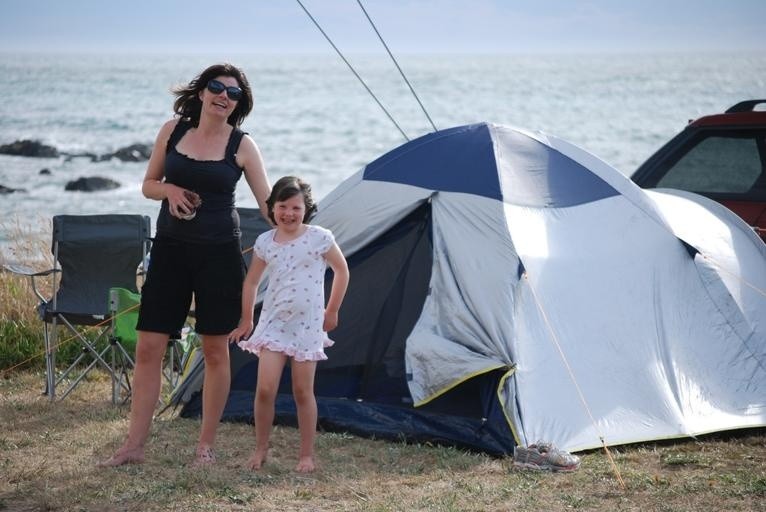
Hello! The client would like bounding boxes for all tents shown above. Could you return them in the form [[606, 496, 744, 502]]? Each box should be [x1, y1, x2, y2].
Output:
[[166, 120, 766, 462]]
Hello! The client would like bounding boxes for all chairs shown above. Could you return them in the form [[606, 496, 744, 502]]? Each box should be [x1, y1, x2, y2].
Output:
[[4, 211, 157, 400], [105, 282, 190, 403], [181, 208, 276, 387]]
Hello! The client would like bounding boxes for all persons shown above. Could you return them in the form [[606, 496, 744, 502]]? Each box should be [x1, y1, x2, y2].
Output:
[[98, 62, 278, 469], [228, 174, 350, 475]]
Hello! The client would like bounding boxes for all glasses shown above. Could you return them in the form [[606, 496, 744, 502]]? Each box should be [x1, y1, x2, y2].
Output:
[[208, 80, 244, 101]]
